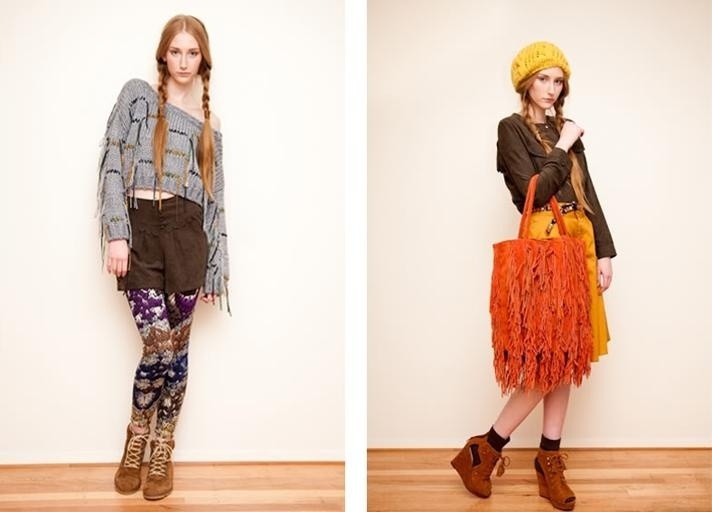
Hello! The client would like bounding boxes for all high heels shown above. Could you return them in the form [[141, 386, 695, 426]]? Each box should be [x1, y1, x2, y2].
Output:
[[450, 435, 511, 499], [534, 447, 577, 511]]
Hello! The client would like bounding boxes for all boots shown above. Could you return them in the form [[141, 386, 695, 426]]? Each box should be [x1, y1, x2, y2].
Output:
[[142, 435, 177, 502], [113, 422, 150, 496]]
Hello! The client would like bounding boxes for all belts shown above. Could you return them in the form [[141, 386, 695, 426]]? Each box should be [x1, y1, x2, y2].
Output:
[[530, 201, 578, 235]]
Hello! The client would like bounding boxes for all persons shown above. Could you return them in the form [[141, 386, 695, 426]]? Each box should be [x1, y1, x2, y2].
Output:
[[95, 14, 232, 500], [447, 43, 616, 510]]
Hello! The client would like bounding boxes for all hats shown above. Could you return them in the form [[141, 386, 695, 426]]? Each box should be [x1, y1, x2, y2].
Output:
[[511, 41, 571, 91]]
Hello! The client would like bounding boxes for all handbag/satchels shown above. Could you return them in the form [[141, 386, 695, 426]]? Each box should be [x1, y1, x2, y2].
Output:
[[487, 173, 596, 398]]
